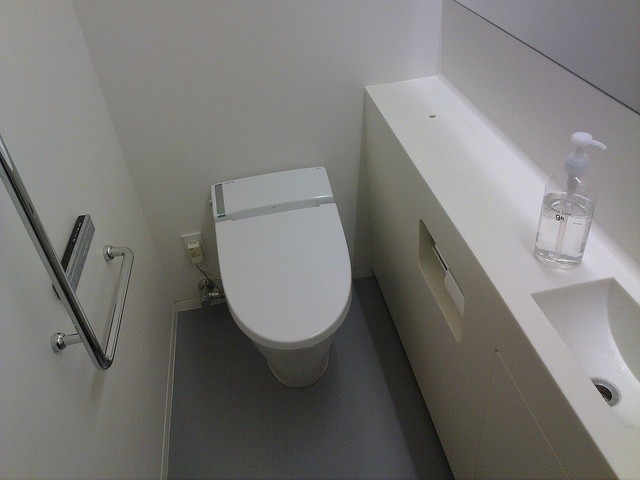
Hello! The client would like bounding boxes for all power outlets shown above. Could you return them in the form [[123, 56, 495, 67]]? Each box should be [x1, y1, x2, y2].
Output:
[[180, 232, 209, 267]]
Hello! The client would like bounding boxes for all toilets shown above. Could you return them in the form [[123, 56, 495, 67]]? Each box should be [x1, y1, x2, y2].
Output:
[[210, 166, 356, 387]]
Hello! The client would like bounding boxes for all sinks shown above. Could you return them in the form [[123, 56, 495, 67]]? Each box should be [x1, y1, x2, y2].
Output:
[[532, 277, 636, 456]]
